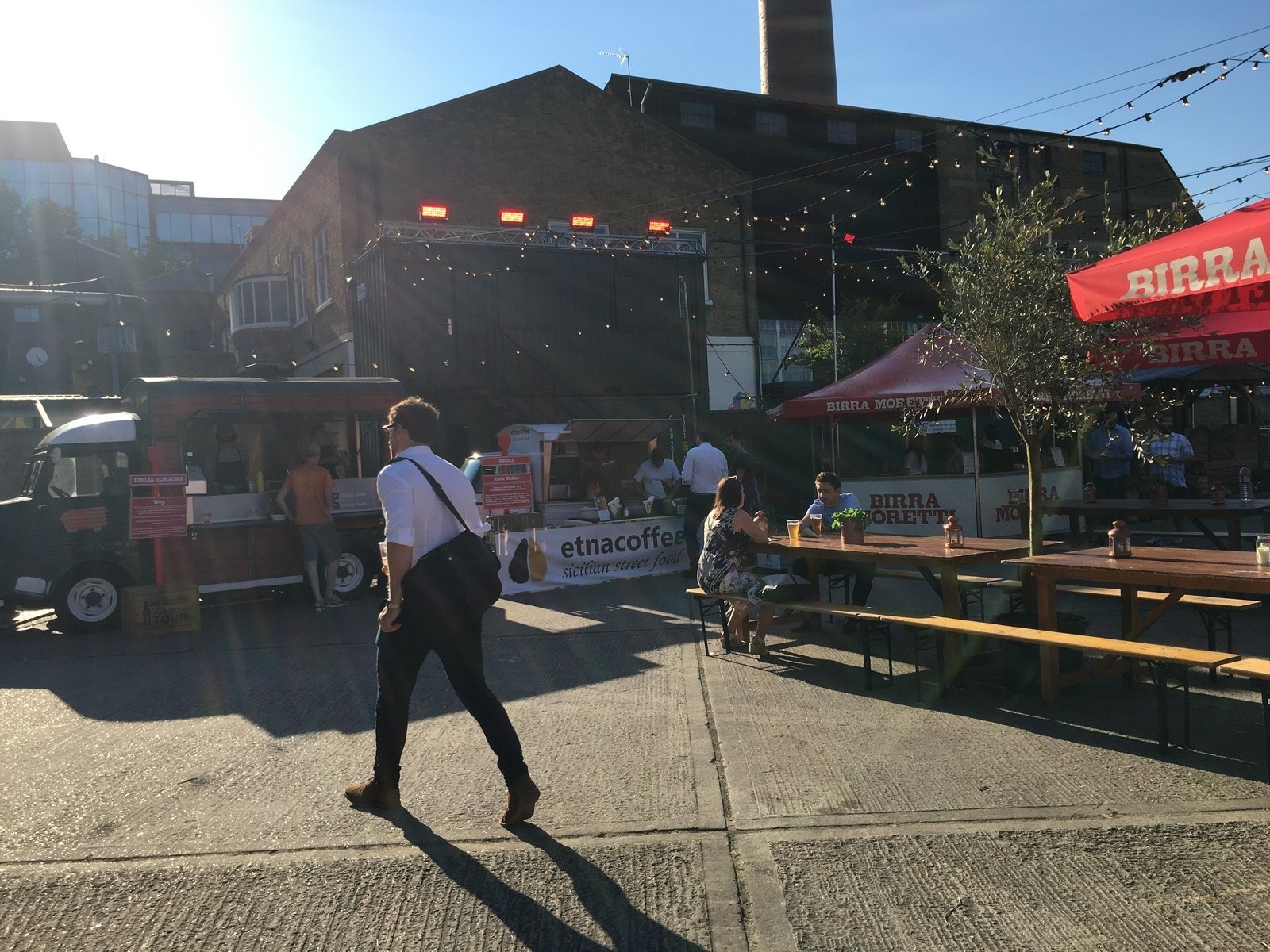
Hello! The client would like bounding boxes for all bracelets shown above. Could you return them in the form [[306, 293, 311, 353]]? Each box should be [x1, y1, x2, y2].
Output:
[[799, 522, 803, 527], [388, 601, 400, 608]]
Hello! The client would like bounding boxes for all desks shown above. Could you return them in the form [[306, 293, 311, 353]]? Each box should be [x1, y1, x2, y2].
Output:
[[749, 534, 1067, 685], [1001, 499, 1270, 551], [1000, 546, 1270, 708]]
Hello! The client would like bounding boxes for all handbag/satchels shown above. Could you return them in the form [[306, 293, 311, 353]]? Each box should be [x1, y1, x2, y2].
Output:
[[405, 529, 503, 636]]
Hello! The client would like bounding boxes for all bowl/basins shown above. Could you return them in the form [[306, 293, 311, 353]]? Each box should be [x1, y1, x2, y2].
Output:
[[579, 508, 599, 520]]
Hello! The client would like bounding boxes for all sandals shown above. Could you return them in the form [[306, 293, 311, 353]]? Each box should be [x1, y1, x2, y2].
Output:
[[748, 634, 770, 657], [719, 632, 741, 649]]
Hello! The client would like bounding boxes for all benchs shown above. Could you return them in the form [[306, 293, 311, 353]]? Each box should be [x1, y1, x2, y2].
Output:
[[686, 531, 1270, 769]]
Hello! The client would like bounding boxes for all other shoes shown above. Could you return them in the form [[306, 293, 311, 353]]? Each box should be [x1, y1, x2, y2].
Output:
[[840, 618, 858, 634], [499, 772, 540, 826], [683, 568, 697, 578], [315, 599, 327, 613], [344, 777, 401, 809], [325, 598, 351, 608], [789, 620, 821, 633]]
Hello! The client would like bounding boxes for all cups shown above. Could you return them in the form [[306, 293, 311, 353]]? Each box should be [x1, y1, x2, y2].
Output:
[[810, 514, 822, 532], [378, 542, 388, 576], [786, 519, 799, 541]]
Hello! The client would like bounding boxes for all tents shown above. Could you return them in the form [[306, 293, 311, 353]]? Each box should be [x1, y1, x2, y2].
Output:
[[1064, 199, 1270, 372], [766, 323, 1141, 537]]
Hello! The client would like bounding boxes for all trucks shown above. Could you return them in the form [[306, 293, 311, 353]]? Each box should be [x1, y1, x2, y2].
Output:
[[459, 417, 683, 539], [0, 376, 406, 635]]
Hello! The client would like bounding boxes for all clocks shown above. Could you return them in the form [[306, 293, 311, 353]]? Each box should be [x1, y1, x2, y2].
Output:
[[25, 347, 48, 367]]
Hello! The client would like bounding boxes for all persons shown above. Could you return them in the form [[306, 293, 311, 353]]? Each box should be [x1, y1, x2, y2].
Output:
[[1084, 403, 1195, 544], [696, 476, 776, 654], [904, 426, 1003, 476], [790, 472, 876, 633], [345, 397, 540, 826], [205, 419, 349, 613], [568, 441, 623, 498], [633, 417, 760, 580]]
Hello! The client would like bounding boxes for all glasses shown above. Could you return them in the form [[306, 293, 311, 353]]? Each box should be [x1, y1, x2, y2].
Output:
[[732, 475, 739, 484], [382, 425, 395, 436]]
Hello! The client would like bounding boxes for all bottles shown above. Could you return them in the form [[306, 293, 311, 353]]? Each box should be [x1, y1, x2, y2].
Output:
[[623, 508, 629, 517], [1239, 465, 1253, 501], [1255, 535, 1270, 565]]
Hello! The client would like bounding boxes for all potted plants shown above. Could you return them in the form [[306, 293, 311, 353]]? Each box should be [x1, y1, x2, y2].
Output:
[[1142, 473, 1175, 502], [831, 507, 872, 544]]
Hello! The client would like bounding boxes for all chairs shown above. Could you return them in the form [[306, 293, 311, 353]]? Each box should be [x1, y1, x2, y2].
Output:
[[100, 468, 128, 493]]
